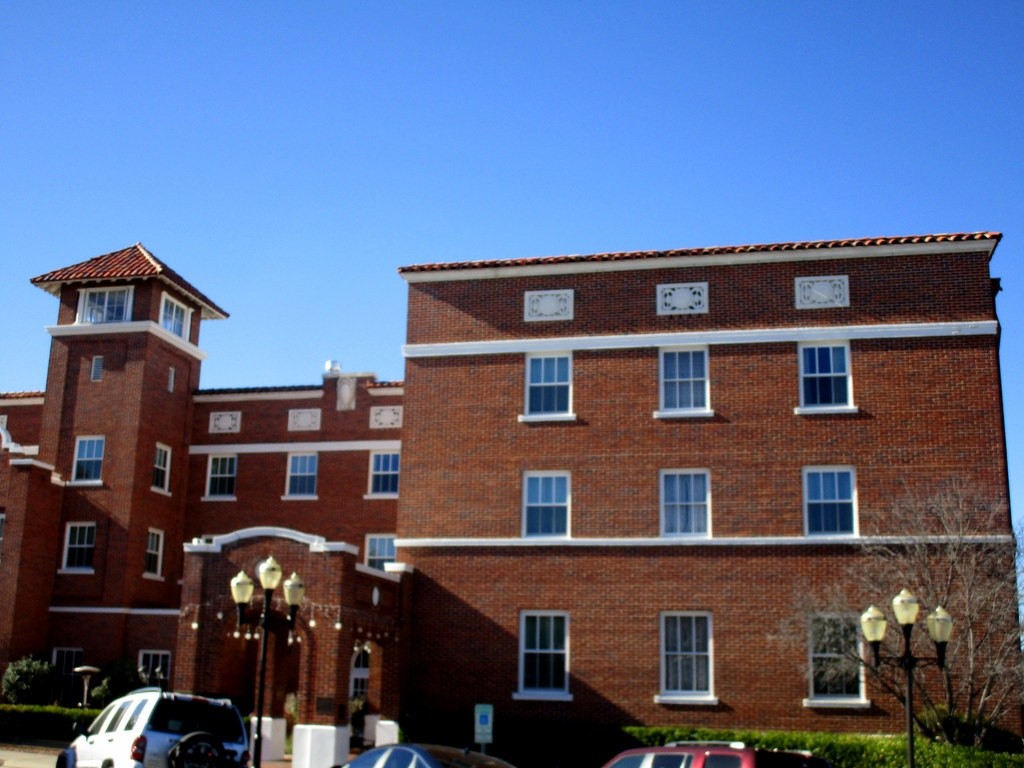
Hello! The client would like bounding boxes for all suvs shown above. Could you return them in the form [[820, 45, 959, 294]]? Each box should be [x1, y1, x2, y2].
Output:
[[230, 552, 307, 768], [56, 685, 250, 768], [597, 739, 818, 768]]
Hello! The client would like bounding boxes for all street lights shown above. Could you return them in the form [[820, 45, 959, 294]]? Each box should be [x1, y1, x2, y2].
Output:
[[858, 588, 953, 768]]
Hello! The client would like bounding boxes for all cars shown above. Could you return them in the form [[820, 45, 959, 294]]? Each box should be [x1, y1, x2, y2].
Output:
[[322, 742, 517, 768]]
